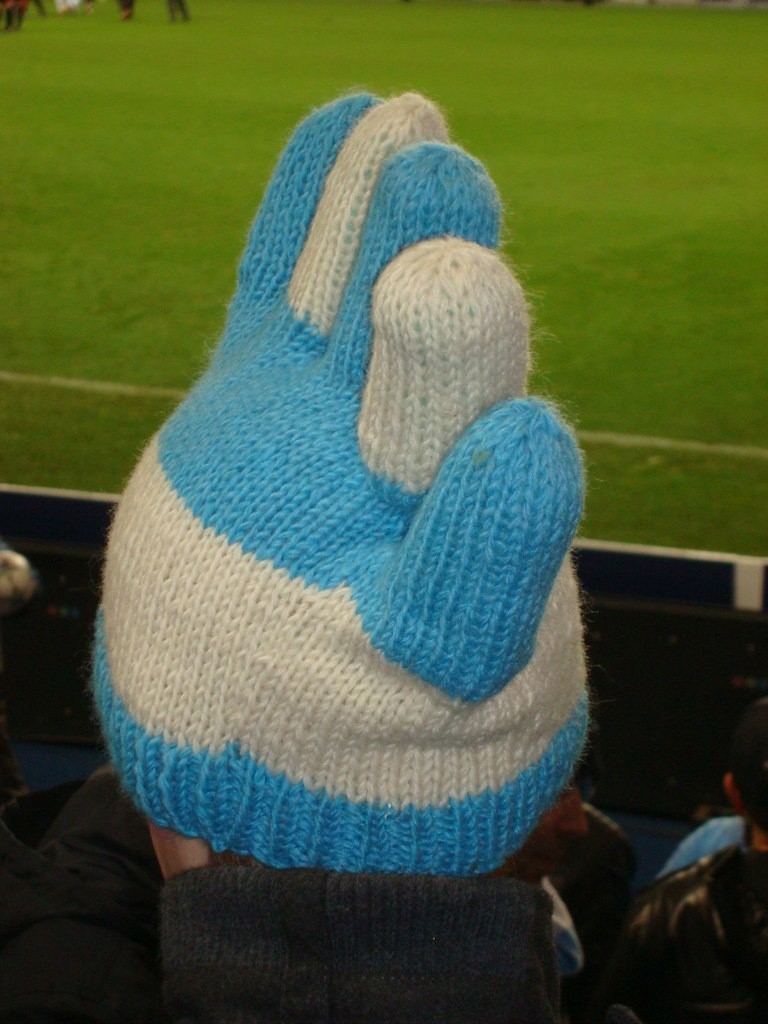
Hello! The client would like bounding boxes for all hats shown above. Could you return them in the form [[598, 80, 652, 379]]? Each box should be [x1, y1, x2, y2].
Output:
[[92, 90, 590, 880]]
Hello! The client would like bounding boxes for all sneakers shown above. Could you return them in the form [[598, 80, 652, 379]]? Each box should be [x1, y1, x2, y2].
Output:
[[2, 25, 13, 32], [13, 25, 21, 32]]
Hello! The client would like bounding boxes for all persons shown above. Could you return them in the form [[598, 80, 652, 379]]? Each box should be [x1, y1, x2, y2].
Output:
[[169, 0, 192, 23], [86, 90, 589, 1024], [119, 0, 135, 20], [0, 763, 163, 1024], [0, 0, 95, 32], [616, 695, 768, 1024], [524, 737, 750, 1024]]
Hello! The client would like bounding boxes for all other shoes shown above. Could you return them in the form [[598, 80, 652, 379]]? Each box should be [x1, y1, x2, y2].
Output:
[[38, 10, 46, 19], [119, 8, 132, 21], [181, 17, 189, 23], [169, 16, 176, 24]]
